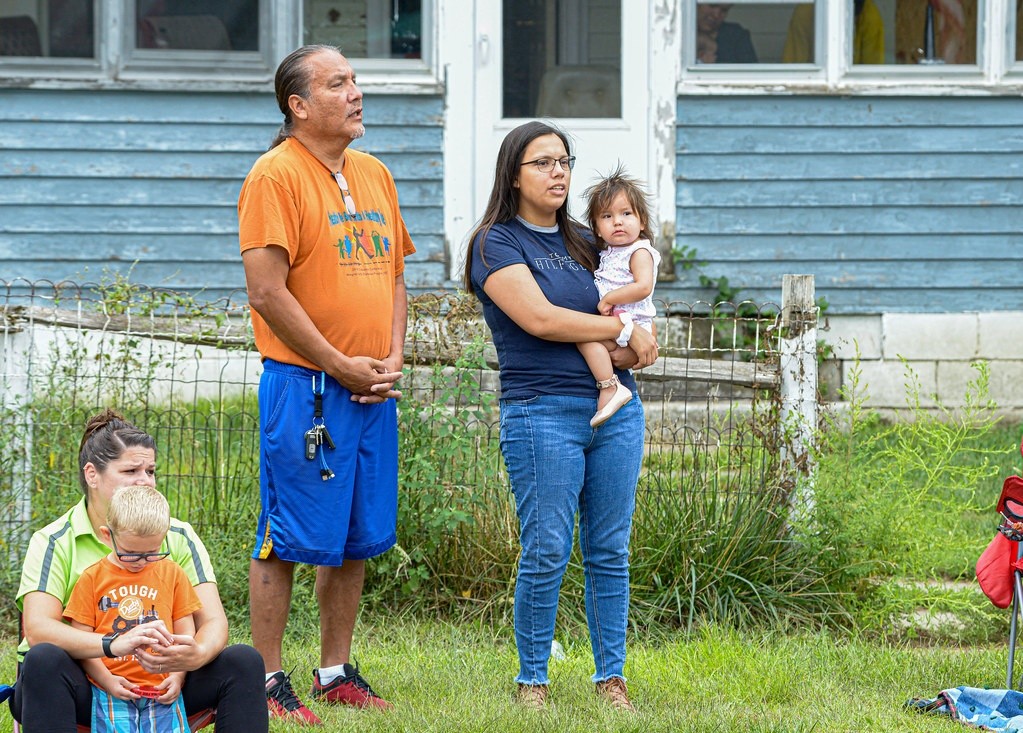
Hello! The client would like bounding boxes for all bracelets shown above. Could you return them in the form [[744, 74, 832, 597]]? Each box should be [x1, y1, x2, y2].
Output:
[[615, 313, 634, 347]]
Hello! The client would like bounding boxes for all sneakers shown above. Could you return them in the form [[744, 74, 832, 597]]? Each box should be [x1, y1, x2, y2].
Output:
[[517, 680, 547, 712], [308, 662, 393, 712], [265, 665, 323, 728], [596, 677, 636, 714]]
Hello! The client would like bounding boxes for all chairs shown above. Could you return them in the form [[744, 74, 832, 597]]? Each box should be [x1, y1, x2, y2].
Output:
[[975, 437, 1023, 690]]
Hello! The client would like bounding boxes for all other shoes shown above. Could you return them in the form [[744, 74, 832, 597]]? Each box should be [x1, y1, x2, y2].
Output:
[[590, 375, 633, 426]]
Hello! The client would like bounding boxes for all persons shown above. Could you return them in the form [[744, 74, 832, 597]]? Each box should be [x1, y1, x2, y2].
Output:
[[9, 409, 271, 732], [697, 4, 757, 64], [783, 0, 884, 65], [464, 120, 660, 715], [236, 42, 416, 728], [695, 32, 718, 64], [575, 158, 662, 427], [62, 486, 201, 733]]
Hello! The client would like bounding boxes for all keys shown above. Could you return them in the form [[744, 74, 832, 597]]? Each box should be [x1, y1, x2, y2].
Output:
[[303, 424, 336, 460]]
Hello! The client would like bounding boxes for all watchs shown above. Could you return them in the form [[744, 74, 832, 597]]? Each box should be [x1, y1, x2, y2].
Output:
[[102, 632, 119, 658]]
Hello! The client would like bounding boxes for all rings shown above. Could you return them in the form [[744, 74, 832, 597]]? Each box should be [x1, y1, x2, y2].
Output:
[[158, 663, 162, 672]]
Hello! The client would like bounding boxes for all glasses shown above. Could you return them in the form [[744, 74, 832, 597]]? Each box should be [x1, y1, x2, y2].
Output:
[[110, 529, 171, 563], [517, 155, 577, 173]]
